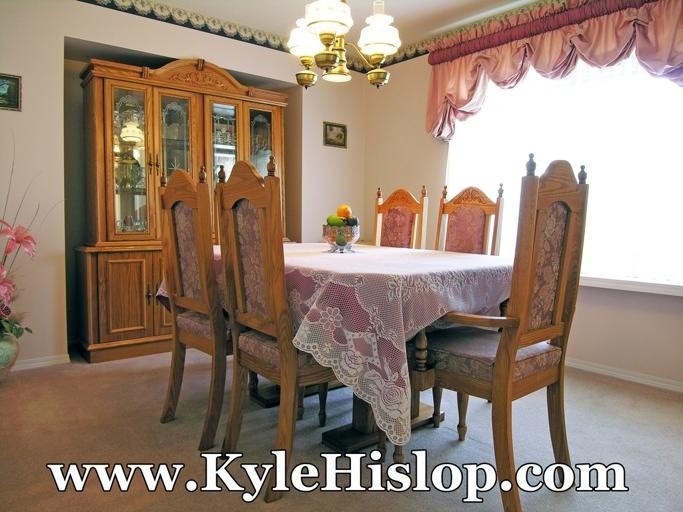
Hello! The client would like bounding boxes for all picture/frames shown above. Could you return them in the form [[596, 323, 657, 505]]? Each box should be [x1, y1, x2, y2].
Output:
[[0, 72, 23, 112], [322, 120, 348, 148]]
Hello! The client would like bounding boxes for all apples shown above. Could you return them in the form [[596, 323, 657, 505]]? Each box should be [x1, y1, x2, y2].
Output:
[[327, 216, 336, 225], [336, 232, 346, 245], [336, 217, 347, 226]]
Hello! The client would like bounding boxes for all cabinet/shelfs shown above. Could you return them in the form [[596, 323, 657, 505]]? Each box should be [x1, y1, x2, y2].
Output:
[[77, 58, 292, 247], [72, 241, 174, 364]]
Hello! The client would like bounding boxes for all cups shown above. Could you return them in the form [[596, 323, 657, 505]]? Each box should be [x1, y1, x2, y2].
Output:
[[115, 215, 143, 233]]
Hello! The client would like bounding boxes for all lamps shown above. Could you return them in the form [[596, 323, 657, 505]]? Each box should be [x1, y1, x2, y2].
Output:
[[285, 0, 404, 89], [119, 102, 144, 143]]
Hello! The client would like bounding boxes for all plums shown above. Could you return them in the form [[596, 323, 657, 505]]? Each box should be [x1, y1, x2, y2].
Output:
[[347, 217, 357, 225]]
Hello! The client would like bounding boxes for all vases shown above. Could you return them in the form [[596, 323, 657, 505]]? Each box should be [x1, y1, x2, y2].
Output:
[[0, 333, 20, 386]]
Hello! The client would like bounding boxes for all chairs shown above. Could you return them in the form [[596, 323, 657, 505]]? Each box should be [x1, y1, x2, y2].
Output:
[[157, 156, 591, 512]]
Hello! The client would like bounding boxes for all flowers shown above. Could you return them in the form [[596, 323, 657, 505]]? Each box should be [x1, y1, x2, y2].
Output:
[[0, 126, 67, 340]]
